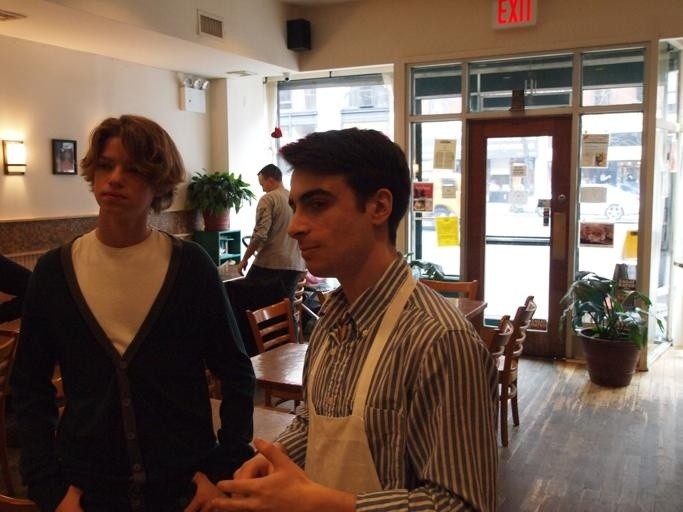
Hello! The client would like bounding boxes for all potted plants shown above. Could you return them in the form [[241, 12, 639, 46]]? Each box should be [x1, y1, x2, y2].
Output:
[[564, 268, 646, 390], [188, 168, 253, 229]]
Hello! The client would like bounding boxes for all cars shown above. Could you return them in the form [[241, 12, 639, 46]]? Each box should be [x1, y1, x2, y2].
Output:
[[524, 184, 639, 221]]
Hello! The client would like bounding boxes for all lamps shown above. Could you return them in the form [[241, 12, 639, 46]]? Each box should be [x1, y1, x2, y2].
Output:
[[1, 137, 32, 175], [181, 76, 209, 114]]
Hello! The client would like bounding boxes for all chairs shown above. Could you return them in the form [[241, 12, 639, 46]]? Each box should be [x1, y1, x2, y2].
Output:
[[488, 315, 515, 366], [499, 296, 536, 446], [419, 277, 479, 300], [317, 291, 327, 305], [1, 330, 19, 492], [245, 297, 301, 413]]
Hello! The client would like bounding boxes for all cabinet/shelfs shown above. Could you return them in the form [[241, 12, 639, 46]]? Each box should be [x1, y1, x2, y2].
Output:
[[199, 229, 242, 266]]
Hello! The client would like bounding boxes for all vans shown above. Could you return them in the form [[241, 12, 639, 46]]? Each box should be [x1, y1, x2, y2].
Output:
[[421, 168, 461, 228]]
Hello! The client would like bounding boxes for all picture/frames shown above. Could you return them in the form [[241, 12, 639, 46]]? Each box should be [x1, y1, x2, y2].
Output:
[[52, 137, 83, 178]]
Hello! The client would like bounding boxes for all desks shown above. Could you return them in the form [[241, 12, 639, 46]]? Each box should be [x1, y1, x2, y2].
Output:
[[250, 296, 487, 407]]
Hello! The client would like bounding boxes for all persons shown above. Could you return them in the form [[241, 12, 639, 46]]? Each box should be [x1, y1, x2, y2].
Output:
[[0, 253, 33, 326], [8, 114, 258, 512], [211, 127, 499, 512], [236, 164, 307, 357]]
